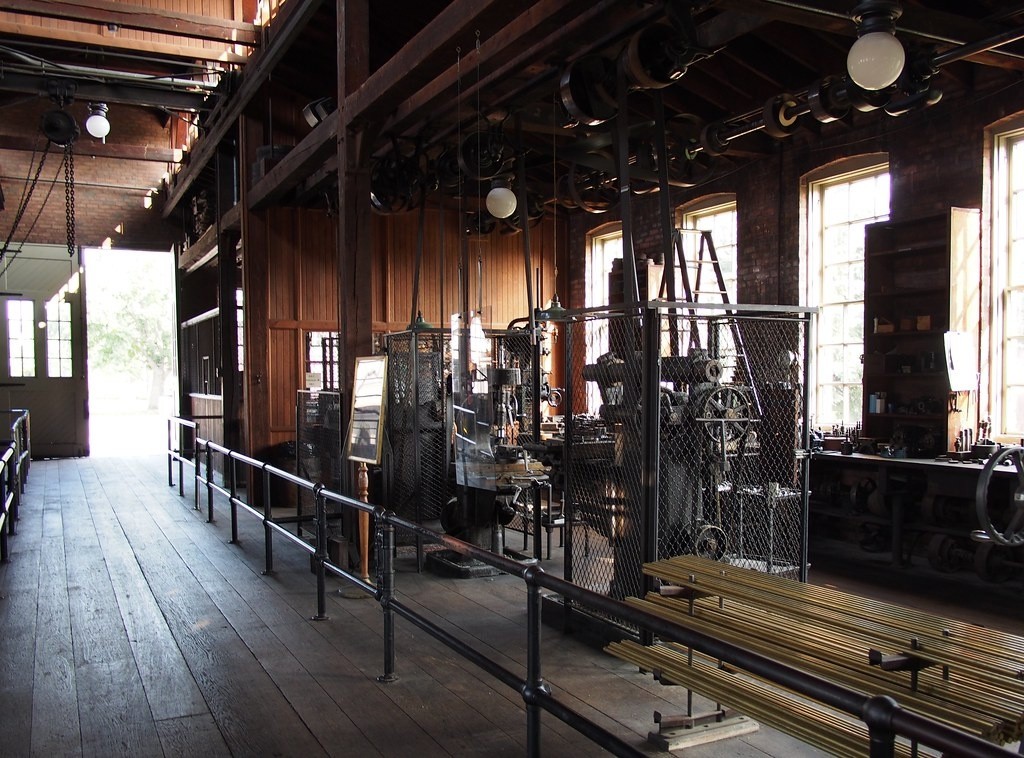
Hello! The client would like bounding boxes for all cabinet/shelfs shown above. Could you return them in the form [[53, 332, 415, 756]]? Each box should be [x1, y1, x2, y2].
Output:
[[861, 210, 950, 458], [810, 449, 1024, 609]]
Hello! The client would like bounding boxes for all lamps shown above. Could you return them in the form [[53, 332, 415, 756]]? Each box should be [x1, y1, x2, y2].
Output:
[[86, 102, 110, 137], [486, 146, 517, 219], [406, 292, 433, 331], [846, 0, 904, 91], [536, 71, 569, 322]]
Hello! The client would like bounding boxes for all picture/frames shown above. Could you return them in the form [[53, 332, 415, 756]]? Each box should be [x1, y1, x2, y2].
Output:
[[344, 356, 389, 465]]
